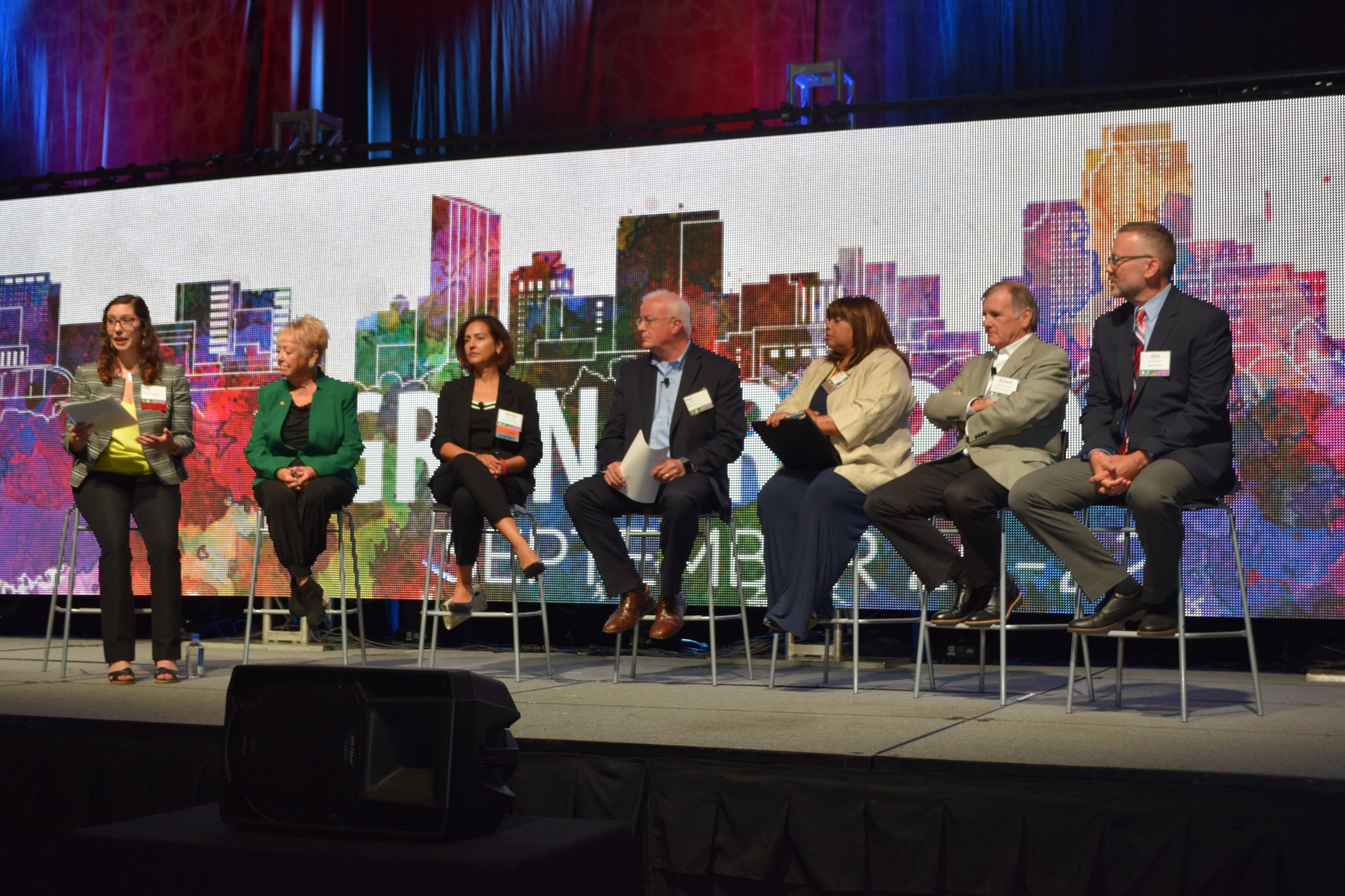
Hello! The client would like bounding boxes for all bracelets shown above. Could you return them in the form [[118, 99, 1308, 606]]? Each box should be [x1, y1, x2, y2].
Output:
[[167, 440, 179, 454], [474, 453, 481, 456], [500, 458, 508, 478]]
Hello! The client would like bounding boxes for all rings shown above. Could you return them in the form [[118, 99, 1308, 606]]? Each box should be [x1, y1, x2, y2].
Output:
[[666, 473, 669, 477]]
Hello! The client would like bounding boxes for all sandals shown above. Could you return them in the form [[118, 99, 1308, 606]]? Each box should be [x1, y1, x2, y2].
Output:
[[108, 659, 135, 684], [153, 657, 181, 683]]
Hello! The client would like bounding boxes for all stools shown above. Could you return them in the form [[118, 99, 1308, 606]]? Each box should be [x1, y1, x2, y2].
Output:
[[242, 502, 367, 666], [614, 472, 1264, 722], [41, 504, 153, 678], [417, 502, 552, 683]]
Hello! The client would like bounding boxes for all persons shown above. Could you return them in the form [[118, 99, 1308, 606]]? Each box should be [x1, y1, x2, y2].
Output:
[[563, 289, 748, 640], [426, 314, 548, 613], [1006, 222, 1238, 638], [60, 295, 195, 686], [243, 313, 365, 631], [863, 279, 1072, 626], [757, 295, 918, 641]]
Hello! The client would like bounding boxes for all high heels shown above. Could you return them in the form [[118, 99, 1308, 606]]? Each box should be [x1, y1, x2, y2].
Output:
[[296, 576, 330, 629], [449, 584, 474, 613], [289, 575, 306, 617], [520, 559, 546, 582]]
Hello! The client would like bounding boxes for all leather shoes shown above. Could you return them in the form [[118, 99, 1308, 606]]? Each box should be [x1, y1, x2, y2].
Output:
[[1068, 586, 1146, 633], [1137, 597, 1179, 636], [965, 575, 1022, 626], [808, 618, 820, 630], [931, 583, 990, 623], [763, 616, 789, 632], [649, 592, 688, 640], [602, 583, 657, 633]]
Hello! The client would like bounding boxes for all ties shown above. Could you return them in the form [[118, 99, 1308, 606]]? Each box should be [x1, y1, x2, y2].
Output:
[[1115, 309, 1147, 455]]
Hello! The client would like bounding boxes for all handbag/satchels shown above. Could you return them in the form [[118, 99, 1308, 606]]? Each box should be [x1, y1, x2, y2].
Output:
[[441, 583, 487, 630]]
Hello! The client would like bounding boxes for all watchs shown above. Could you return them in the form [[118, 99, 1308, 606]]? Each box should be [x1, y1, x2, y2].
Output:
[[679, 457, 693, 474]]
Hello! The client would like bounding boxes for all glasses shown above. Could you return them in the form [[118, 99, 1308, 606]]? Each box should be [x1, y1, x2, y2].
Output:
[[1106, 254, 1155, 265], [103, 316, 141, 329], [634, 315, 676, 328]]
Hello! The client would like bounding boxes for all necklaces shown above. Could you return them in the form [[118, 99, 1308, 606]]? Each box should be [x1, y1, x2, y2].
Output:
[[121, 368, 138, 382], [296, 383, 313, 394]]
